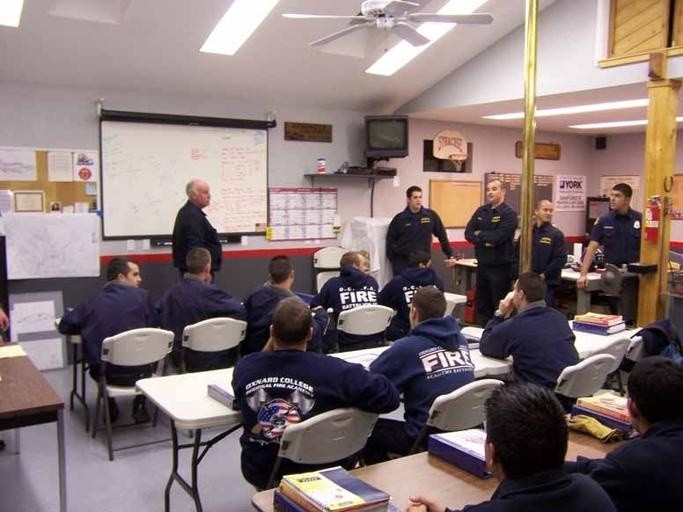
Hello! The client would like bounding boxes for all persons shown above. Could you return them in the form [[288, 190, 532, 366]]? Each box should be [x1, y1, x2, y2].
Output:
[[310, 252, 383, 350], [0, 305, 10, 454], [564, 356, 683, 510], [405, 378, 615, 511], [376, 251, 444, 341], [58, 258, 156, 425], [479, 271, 578, 390], [241, 255, 330, 357], [516, 197, 567, 306], [362, 284, 475, 466], [172, 181, 223, 284], [230, 295, 398, 490], [576, 183, 641, 315], [464, 181, 518, 326], [385, 183, 454, 274], [154, 248, 247, 370]]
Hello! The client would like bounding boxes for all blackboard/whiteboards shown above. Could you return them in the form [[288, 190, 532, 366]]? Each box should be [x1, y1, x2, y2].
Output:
[[99, 119, 270, 241], [2, 211, 101, 281]]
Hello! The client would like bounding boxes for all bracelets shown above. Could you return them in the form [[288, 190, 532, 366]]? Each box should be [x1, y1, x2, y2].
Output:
[[448, 256, 453, 259], [493, 309, 504, 317]]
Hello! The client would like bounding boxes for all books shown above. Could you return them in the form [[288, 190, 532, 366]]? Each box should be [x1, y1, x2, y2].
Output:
[[574, 312, 626, 326], [425, 427, 493, 480], [207, 380, 238, 412], [576, 393, 632, 423], [573, 320, 627, 335], [571, 405, 635, 439], [276, 465, 392, 510], [272, 487, 399, 512], [460, 326, 485, 349]]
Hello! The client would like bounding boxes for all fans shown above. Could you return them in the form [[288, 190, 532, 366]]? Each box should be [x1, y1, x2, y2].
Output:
[[282, 0, 498, 55]]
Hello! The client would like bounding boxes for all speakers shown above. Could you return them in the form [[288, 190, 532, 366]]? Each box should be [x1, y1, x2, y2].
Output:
[[595, 137, 606, 149]]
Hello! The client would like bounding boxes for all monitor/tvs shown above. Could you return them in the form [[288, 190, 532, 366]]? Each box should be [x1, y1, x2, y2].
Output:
[[364, 114, 410, 157]]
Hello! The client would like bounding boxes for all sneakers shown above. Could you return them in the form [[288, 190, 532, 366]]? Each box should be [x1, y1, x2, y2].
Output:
[[132, 405, 150, 423], [102, 398, 118, 422]]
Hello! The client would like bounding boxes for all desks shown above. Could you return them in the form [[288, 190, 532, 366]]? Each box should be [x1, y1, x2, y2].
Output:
[[252, 412, 637, 511], [1, 338, 69, 511], [54, 290, 466, 434], [134, 314, 648, 512]]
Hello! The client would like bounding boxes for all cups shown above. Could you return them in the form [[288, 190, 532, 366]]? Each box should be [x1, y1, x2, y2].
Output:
[[317, 157, 325, 174]]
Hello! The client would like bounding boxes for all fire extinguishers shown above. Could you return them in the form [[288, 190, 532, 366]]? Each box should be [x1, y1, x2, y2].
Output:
[[644, 195, 660, 244]]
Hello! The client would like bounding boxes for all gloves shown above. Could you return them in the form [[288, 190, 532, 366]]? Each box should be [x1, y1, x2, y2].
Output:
[[568, 414, 624, 443]]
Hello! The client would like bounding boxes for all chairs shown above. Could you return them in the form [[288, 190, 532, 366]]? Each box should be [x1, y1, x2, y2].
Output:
[[92, 328, 176, 457], [261, 298, 457, 356], [263, 318, 674, 487], [153, 316, 248, 441]]
